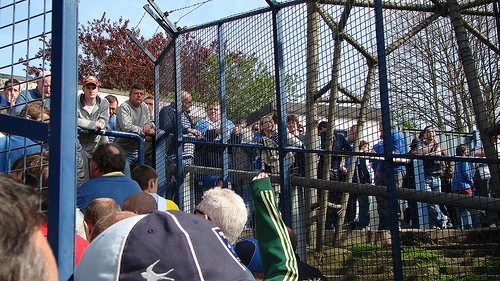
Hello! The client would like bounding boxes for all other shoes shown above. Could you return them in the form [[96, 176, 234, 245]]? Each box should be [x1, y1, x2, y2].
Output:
[[433, 215, 454, 230]]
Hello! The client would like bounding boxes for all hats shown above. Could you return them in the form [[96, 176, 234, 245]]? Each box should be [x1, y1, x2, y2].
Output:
[[408, 138, 422, 147], [82, 76, 99, 87], [230, 237, 264, 272], [72, 209, 257, 281]]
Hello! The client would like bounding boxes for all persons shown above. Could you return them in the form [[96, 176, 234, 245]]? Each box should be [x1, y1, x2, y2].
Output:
[[317, 118, 500, 230], [0, 71, 325, 281]]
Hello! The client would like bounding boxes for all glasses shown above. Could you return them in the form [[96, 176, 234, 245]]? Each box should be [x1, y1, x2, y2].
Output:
[[193, 209, 211, 221]]
